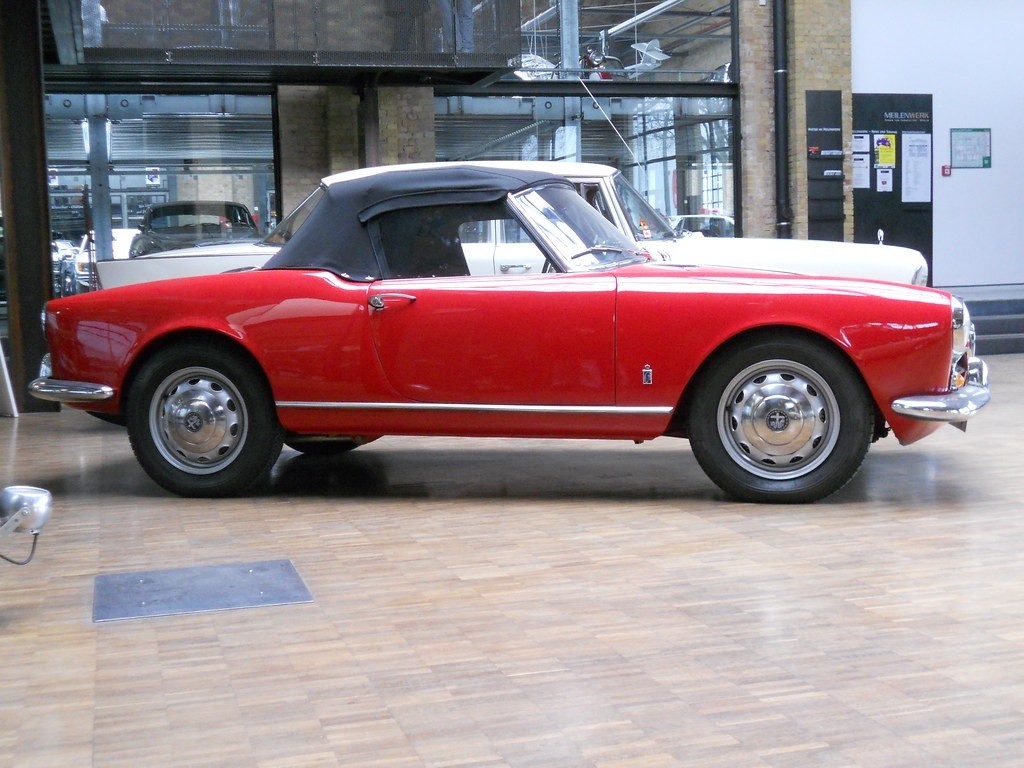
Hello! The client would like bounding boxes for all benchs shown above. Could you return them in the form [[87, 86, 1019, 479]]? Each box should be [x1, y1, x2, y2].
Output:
[[387, 219, 470, 277]]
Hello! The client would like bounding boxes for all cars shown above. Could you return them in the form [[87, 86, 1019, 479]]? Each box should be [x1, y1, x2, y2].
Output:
[[129, 199, 258, 257], [26, 164, 990, 504], [73, 227, 142, 296], [94, 161, 930, 287], [51, 238, 73, 294], [652, 215, 734, 238]]
